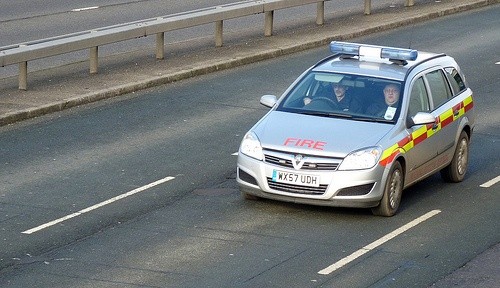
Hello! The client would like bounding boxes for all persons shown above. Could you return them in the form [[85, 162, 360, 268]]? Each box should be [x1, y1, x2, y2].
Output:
[[303, 83, 362, 113], [365, 83, 400, 121]]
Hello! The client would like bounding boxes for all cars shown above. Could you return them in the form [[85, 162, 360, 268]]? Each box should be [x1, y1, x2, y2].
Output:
[[236, 41, 475, 216]]
